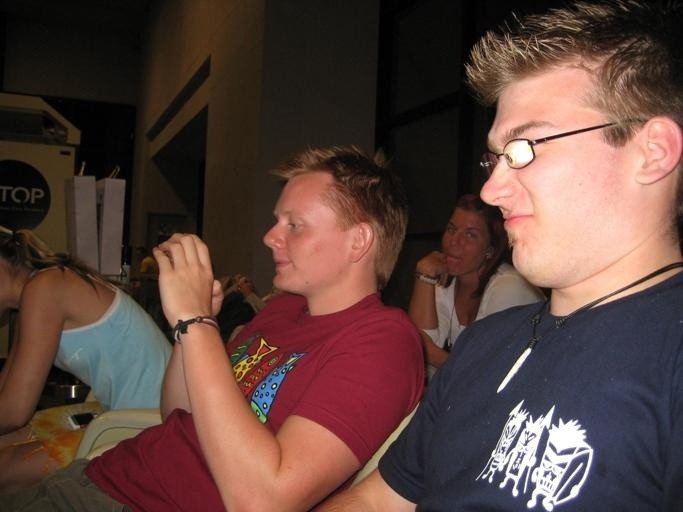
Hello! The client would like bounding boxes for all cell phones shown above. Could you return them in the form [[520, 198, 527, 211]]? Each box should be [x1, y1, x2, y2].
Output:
[[68, 411, 98, 430]]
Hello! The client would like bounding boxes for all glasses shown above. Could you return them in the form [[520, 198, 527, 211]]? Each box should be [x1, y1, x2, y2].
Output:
[[476, 115, 652, 178]]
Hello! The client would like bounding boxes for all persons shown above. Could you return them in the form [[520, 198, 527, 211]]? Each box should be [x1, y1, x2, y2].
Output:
[[311, 1, 683, 511], [2, 143, 547, 512]]
